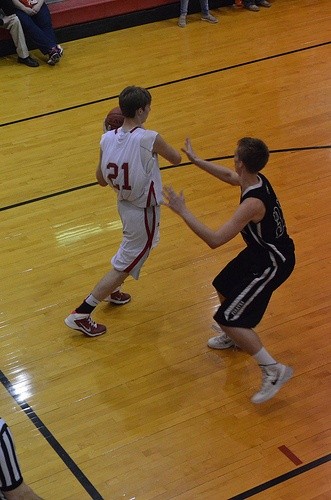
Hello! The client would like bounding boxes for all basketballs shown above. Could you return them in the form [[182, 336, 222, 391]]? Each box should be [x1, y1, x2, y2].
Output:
[[105, 107, 124, 131]]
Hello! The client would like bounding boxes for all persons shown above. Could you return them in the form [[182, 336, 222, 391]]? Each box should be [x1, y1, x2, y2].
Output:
[[178, 0, 219, 28], [244, 0, 272, 12], [159, 136, 296, 404], [0, 416, 46, 500], [63, 84, 183, 337], [0, 0, 40, 67], [12, 0, 64, 65]]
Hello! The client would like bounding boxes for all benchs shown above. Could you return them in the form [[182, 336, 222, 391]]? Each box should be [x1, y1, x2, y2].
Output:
[[0, 0, 180, 40]]
